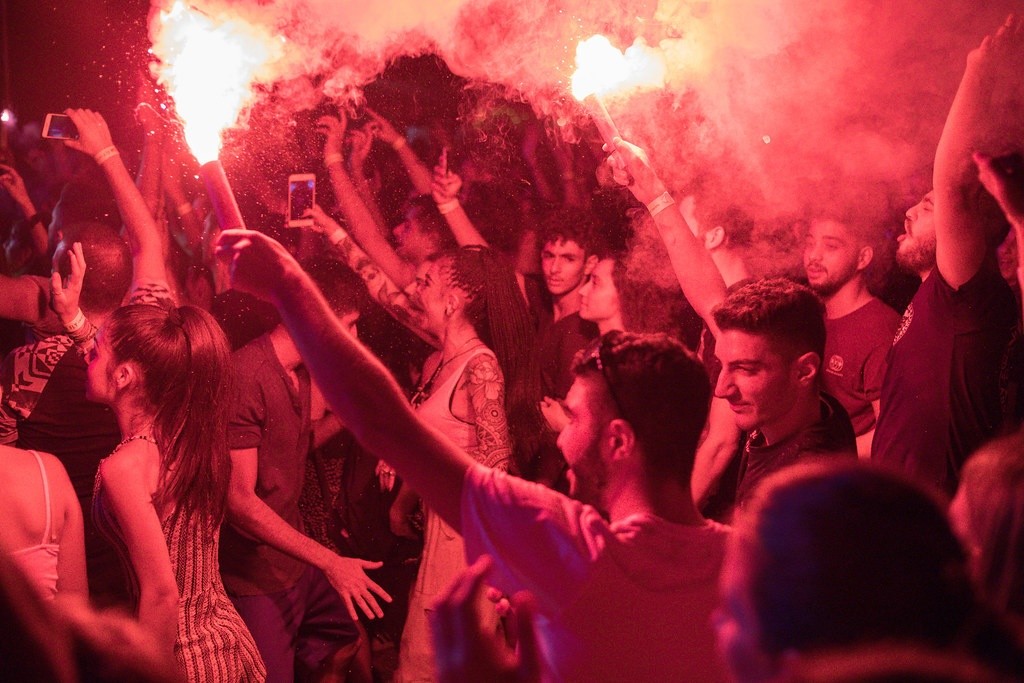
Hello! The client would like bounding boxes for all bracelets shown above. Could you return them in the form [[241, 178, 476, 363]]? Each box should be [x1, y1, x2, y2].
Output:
[[646, 191, 675, 218], [177, 202, 192, 216], [25, 212, 47, 233], [328, 227, 348, 245], [69, 322, 99, 344], [392, 136, 407, 151], [62, 308, 85, 333], [93, 145, 120, 166], [324, 152, 344, 167], [438, 198, 460, 214]]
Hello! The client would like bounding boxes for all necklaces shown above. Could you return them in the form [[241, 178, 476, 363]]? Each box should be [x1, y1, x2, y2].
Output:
[[411, 336, 488, 409]]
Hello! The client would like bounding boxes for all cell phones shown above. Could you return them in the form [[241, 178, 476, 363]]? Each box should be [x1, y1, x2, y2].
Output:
[[42, 114, 79, 140], [288, 174, 316, 227]]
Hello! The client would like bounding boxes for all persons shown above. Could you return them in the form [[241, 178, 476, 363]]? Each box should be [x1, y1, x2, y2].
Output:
[[0, 12, 1024, 683]]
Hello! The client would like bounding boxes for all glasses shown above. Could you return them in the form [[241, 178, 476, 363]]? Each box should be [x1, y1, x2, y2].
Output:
[[582, 330, 640, 442]]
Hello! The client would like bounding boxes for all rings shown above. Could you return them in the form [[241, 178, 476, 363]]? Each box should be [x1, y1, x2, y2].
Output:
[[50, 292, 63, 295]]
